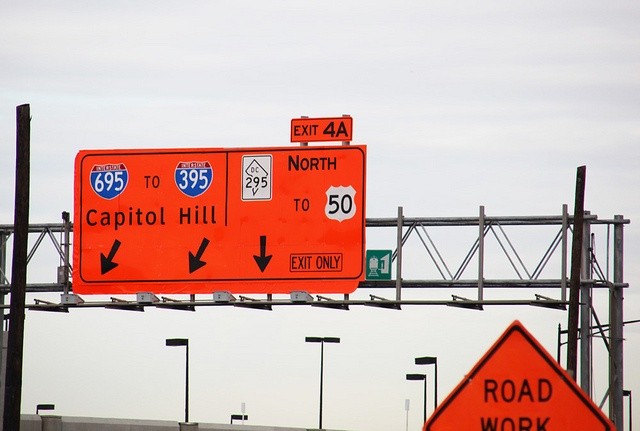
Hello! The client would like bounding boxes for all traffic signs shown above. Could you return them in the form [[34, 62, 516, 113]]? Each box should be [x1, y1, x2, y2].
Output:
[[72, 145, 369, 295], [292, 118, 352, 142], [417, 317, 610, 431], [365, 248, 392, 281]]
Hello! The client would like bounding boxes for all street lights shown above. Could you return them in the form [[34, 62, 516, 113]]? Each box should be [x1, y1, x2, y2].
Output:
[[305, 336, 340, 430], [414, 355, 438, 411], [407, 373, 427, 431], [230, 415, 248, 426], [33, 404, 55, 417], [165, 338, 189, 424]]
